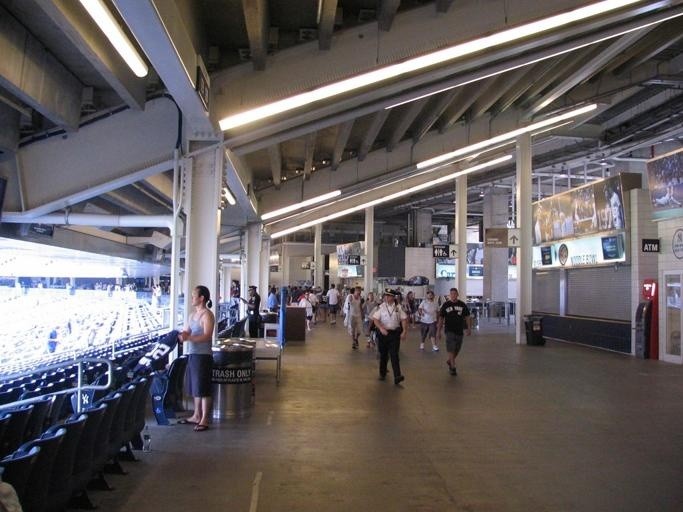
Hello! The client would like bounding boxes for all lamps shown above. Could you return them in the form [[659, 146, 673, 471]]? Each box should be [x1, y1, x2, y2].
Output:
[[261, 187, 341, 222], [224, 188, 236, 206], [270, 154, 513, 239], [414, 104, 600, 170], [217, 1, 643, 133], [77, 0, 150, 79]]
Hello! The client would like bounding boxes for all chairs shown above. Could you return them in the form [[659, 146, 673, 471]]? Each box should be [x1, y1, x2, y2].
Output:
[[0, 306, 250, 512]]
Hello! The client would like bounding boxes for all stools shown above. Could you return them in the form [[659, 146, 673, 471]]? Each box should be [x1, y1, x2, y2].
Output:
[[263, 323, 279, 341]]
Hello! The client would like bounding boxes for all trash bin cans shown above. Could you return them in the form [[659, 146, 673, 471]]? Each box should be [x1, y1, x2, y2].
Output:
[[213, 338, 256, 406], [468, 306, 480, 329], [212, 344, 252, 420], [524, 315, 545, 346]]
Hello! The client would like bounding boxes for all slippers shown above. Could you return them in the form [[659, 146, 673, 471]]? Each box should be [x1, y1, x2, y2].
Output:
[[193, 425, 209, 431], [177, 419, 198, 424]]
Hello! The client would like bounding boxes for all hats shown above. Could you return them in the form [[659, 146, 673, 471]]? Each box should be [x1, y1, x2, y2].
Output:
[[248, 286, 257, 290], [384, 288, 398, 296]]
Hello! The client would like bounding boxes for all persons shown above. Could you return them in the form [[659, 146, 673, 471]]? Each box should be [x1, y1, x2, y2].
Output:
[[243, 286, 261, 338], [374, 288, 407, 384], [95, 281, 169, 305], [47, 325, 59, 353], [534, 180, 623, 243], [653, 152, 683, 207], [419, 288, 471, 377], [178, 286, 215, 431], [267, 284, 419, 349]]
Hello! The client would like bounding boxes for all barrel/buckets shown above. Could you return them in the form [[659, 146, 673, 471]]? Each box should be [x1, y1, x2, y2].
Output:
[[487, 303, 498, 317], [211, 338, 256, 421]]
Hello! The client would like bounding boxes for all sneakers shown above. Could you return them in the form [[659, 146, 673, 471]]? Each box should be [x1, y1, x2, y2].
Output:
[[433, 346, 439, 351], [380, 376, 385, 380], [352, 339, 358, 349], [421, 343, 424, 349], [394, 376, 404, 384], [447, 359, 457, 376]]
